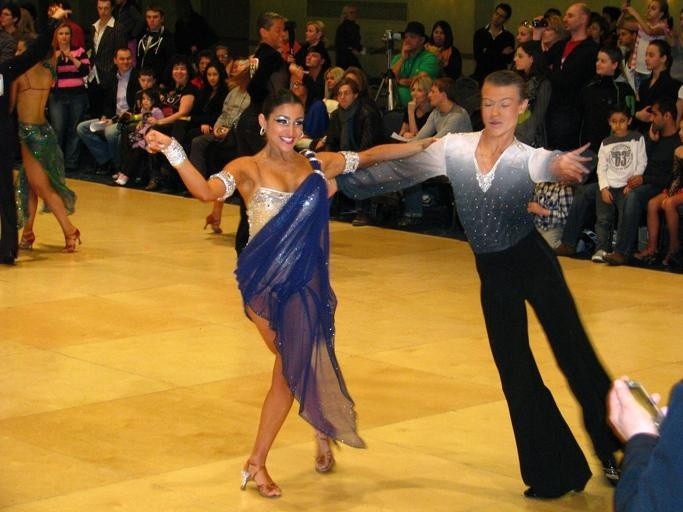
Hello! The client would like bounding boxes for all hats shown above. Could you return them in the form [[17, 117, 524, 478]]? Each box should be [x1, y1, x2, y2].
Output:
[[401, 20, 430, 46]]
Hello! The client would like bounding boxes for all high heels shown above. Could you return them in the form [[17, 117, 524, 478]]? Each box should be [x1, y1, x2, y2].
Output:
[[60, 227, 84, 253], [19, 231, 37, 252], [314, 422, 342, 473], [631, 247, 658, 265], [241, 456, 284, 499], [202, 214, 223, 234]]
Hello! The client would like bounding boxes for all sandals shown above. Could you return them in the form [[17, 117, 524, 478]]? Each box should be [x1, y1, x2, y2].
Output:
[[661, 251, 679, 266]]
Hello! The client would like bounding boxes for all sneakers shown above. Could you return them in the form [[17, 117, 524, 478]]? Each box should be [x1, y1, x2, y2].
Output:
[[352, 212, 373, 225], [95, 168, 191, 196], [396, 216, 423, 227], [552, 244, 578, 260], [601, 252, 625, 266], [591, 249, 607, 263]]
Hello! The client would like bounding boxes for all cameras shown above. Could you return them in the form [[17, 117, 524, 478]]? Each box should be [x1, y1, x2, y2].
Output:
[[532, 19, 548, 27], [383, 30, 401, 42]]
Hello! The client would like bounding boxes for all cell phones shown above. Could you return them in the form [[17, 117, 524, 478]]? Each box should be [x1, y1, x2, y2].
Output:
[[624, 377, 665, 429]]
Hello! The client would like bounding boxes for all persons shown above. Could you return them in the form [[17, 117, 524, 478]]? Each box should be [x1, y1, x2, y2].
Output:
[[144, 87, 444, 498], [607, 375, 682, 507], [325, 70, 629, 499]]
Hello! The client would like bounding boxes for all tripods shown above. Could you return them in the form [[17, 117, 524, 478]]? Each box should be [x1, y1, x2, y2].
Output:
[[374, 47, 402, 112]]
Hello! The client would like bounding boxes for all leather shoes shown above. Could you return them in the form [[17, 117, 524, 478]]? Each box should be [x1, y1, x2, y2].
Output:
[[524, 475, 590, 499], [0, 247, 19, 262], [604, 451, 625, 486]]
